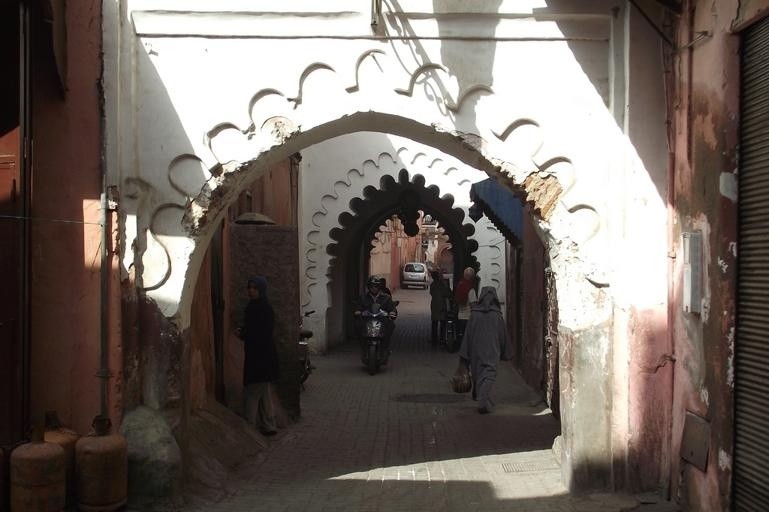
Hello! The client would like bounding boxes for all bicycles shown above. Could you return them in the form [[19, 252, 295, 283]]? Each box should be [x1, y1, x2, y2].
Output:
[[299, 310, 316, 392]]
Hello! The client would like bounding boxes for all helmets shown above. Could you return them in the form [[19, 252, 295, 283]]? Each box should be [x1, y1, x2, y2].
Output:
[[367, 275, 386, 289]]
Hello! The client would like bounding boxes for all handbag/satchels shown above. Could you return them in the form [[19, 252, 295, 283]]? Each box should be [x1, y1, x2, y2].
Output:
[[449, 373, 472, 393]]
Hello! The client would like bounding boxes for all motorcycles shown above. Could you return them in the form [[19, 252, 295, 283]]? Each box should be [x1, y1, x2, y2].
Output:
[[439, 291, 463, 353], [356, 300, 400, 376]]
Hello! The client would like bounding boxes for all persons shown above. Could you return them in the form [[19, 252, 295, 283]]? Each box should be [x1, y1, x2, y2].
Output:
[[429, 267, 514, 414], [351, 277, 398, 338], [234, 276, 276, 434]]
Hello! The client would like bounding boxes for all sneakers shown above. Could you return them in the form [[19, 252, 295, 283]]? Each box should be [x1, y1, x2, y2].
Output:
[[472, 390, 488, 413], [260, 431, 276, 436]]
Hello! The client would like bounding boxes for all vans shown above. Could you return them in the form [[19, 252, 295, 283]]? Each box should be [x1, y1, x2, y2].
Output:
[[402, 263, 430, 290]]
